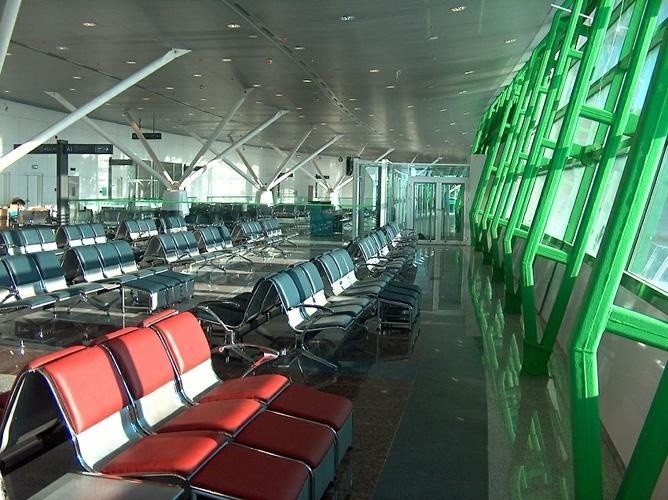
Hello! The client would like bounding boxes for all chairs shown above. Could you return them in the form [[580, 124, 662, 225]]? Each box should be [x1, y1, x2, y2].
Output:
[[1, 204, 422, 500]]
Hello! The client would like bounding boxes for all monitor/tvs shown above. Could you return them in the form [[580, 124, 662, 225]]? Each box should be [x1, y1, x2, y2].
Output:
[[345, 155, 354, 176]]
